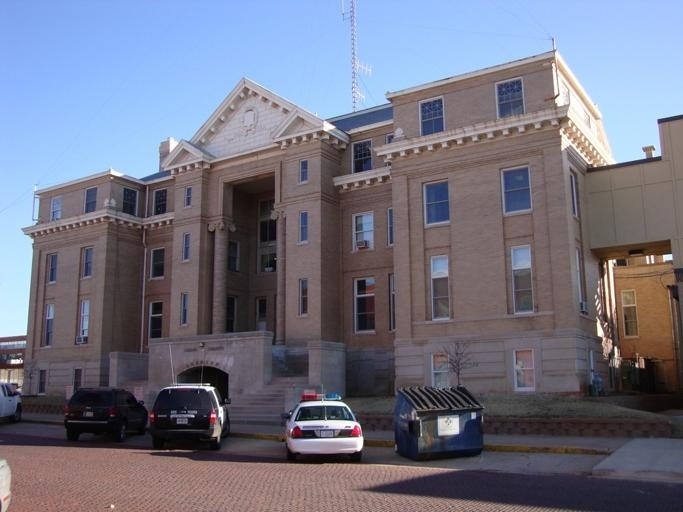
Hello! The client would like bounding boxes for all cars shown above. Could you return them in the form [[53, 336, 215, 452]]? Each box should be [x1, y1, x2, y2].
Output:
[[0, 458, 16, 512], [275, 392, 366, 463], [0, 381, 24, 422]]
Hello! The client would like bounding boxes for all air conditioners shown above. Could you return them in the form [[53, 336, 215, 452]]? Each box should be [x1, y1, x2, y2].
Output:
[[355, 239, 367, 248], [75, 335, 87, 344]]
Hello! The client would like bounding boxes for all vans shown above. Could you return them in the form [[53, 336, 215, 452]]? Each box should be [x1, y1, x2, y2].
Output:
[[62, 385, 150, 443]]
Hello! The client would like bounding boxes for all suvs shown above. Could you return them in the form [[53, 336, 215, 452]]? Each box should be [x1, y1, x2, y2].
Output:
[[144, 381, 233, 452]]
